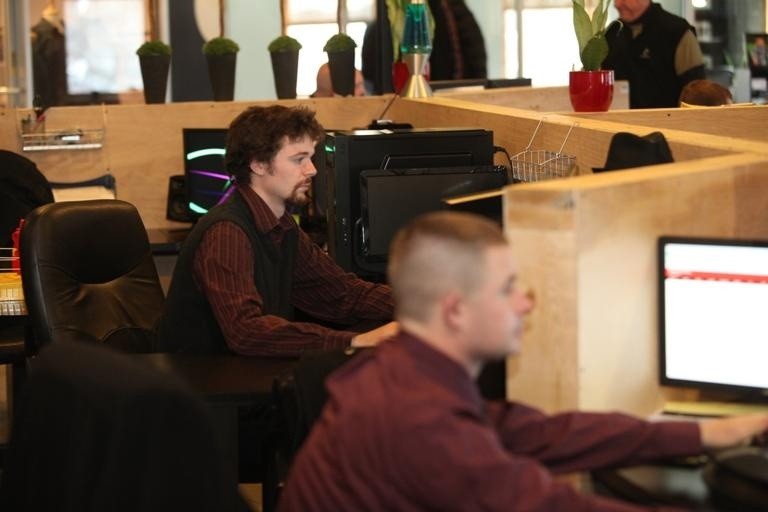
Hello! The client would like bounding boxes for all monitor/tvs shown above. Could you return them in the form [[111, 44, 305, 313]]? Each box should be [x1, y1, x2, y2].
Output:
[[655, 233, 768, 400], [181, 128, 229, 223], [358, 164, 510, 266]]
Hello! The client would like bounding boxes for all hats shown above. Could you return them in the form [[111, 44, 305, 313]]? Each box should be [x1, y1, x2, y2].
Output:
[[591, 132, 675, 172]]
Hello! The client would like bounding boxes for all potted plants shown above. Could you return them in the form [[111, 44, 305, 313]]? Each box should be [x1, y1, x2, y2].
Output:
[[568, 0, 617, 112], [204, 36, 239, 102], [267, 35, 302, 98], [135, 40, 171, 105], [324, 31, 357, 97], [388, 2, 436, 99]]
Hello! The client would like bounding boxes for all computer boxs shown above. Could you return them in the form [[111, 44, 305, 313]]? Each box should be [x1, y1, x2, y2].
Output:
[[336, 128, 494, 284], [301, 137, 325, 248], [325, 133, 338, 263]]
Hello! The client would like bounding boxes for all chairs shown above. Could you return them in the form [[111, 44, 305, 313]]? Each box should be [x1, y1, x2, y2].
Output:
[[2, 149, 57, 365], [17, 197, 285, 511]]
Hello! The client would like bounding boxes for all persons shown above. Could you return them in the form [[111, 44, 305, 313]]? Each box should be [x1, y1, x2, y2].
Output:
[[680, 81, 736, 106], [309, 64, 366, 97], [155, 105, 400, 512], [276, 212, 767, 511], [601, 0, 708, 109], [363, 1, 487, 97]]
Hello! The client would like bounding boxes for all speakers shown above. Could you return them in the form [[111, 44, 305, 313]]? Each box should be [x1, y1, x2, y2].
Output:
[[165, 174, 199, 223]]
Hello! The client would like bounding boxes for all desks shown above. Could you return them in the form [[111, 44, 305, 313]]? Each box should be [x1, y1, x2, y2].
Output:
[[506, 153, 768, 494], [98, 98, 734, 244]]
